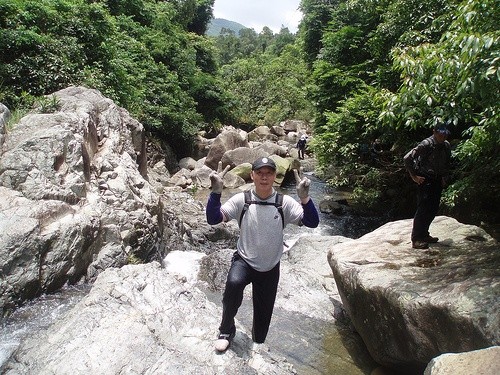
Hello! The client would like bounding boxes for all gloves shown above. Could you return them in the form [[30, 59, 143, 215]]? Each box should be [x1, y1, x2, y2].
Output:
[[209, 161, 230, 193], [293, 167, 311, 198]]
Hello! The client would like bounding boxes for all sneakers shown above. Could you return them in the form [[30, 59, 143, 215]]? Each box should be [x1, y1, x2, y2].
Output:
[[215, 332, 236, 351]]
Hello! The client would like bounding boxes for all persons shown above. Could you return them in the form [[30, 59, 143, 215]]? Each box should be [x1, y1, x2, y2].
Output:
[[403, 126, 453, 248], [206, 156, 319, 350], [296, 136, 306, 160]]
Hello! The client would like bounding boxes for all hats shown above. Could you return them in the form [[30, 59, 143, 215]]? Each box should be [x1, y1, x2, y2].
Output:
[[437, 126, 451, 135], [252, 157, 276, 172]]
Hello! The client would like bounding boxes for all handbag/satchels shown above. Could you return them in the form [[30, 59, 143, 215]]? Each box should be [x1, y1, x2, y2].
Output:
[[409, 170, 433, 190]]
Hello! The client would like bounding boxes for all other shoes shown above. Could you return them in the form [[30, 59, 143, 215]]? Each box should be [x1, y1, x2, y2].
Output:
[[424, 232, 438, 242], [412, 239, 429, 249]]
